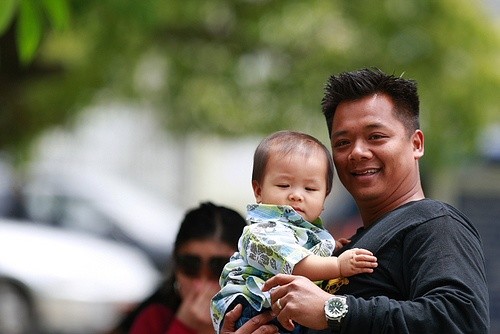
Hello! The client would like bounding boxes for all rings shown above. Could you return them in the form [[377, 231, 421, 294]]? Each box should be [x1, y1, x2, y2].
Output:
[[276, 298, 282, 310]]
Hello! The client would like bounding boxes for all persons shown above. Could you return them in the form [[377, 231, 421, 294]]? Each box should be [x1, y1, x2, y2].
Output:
[[210, 130, 378, 334], [220, 67, 491, 334], [113, 202, 248, 334]]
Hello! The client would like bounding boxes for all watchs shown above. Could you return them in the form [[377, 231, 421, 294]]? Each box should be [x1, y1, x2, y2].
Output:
[[323, 294, 348, 329]]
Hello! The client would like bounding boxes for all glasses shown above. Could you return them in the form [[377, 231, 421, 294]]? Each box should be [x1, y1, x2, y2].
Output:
[[175, 254, 230, 281]]
